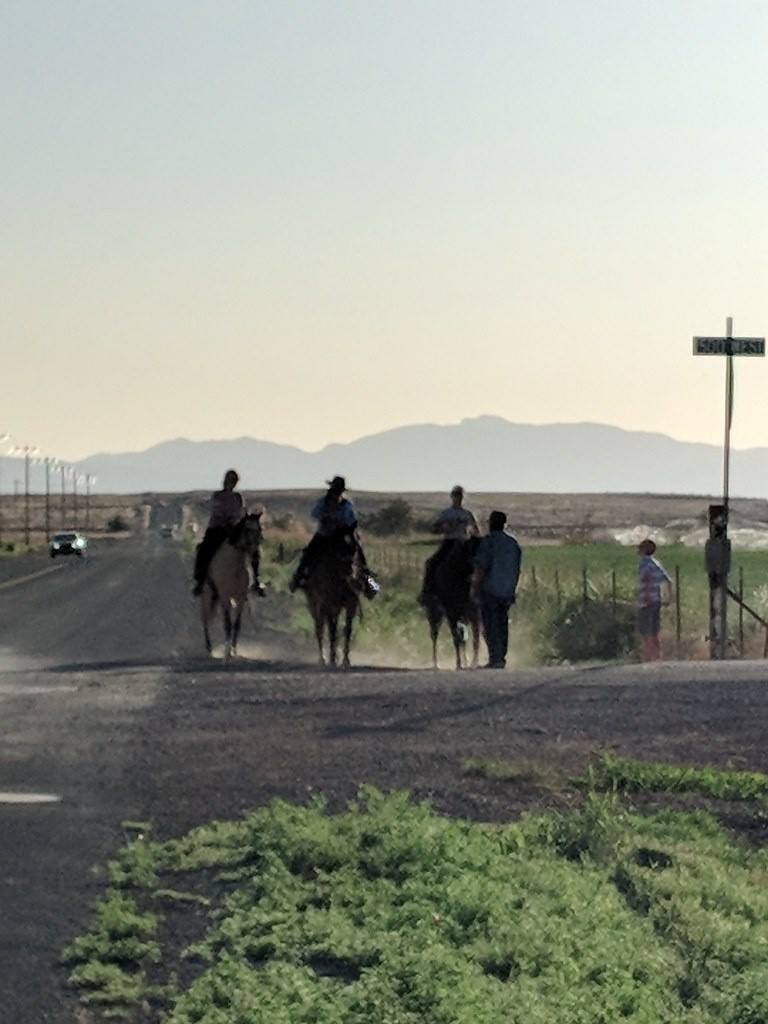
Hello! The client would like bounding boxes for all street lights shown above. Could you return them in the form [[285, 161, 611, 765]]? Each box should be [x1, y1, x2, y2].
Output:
[[82, 473, 95, 530], [67, 468, 81, 532], [52, 462, 72, 533], [8, 445, 39, 547], [32, 455, 56, 541]]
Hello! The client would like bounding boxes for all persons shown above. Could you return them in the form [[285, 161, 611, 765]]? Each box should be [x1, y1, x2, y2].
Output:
[[638, 539, 672, 664], [294, 476, 362, 591], [469, 510, 522, 670], [416, 486, 481, 601], [193, 471, 265, 594]]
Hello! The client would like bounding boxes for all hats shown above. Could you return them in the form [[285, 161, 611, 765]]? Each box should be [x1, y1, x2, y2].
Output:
[[490, 511, 507, 523], [329, 477, 347, 491]]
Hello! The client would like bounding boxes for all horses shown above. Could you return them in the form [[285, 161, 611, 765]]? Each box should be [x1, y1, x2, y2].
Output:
[[196, 506, 269, 658], [299, 519, 370, 668], [415, 534, 479, 668]]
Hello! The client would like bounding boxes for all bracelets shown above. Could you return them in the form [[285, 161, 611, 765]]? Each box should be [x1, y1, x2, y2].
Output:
[[470, 575, 480, 589]]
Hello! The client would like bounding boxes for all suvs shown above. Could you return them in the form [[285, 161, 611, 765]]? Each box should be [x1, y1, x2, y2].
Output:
[[48, 533, 84, 557]]
